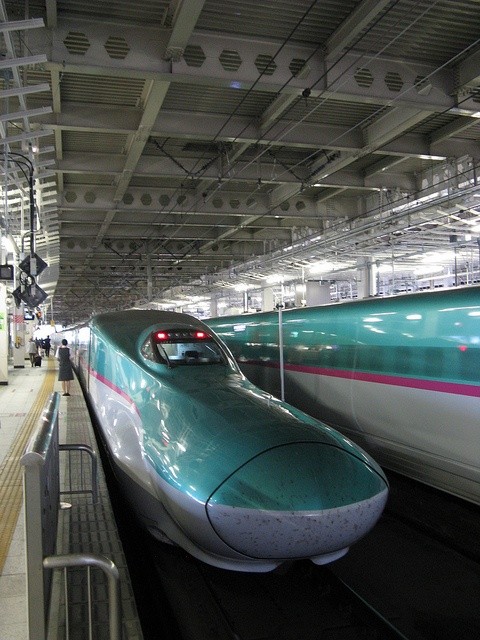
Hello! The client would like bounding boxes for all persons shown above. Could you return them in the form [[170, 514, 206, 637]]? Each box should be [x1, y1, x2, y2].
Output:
[[28, 337, 38, 367], [35, 334, 50, 357], [55, 339, 74, 397]]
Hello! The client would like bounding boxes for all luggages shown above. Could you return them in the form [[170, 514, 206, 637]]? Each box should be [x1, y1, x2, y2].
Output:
[[33, 352, 43, 367]]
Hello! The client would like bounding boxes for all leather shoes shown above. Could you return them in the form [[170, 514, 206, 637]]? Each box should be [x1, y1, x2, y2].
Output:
[[62, 392, 65, 397], [67, 393, 69, 395]]
